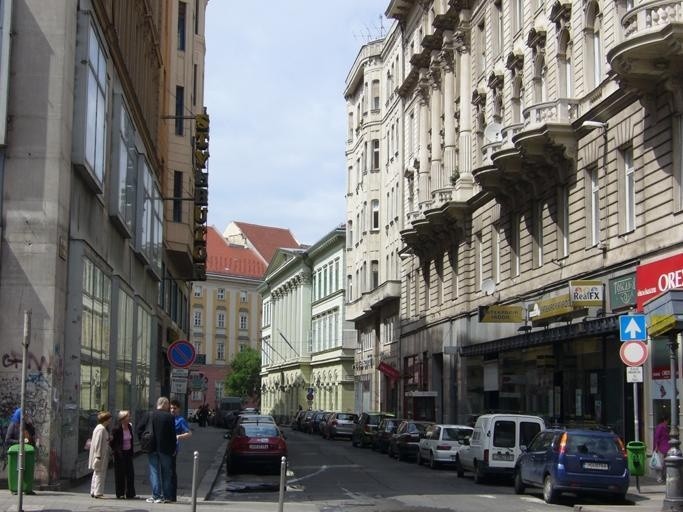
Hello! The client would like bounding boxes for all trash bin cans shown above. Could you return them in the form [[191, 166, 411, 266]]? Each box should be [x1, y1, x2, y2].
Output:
[[7, 444, 35, 494], [626, 441, 647, 476]]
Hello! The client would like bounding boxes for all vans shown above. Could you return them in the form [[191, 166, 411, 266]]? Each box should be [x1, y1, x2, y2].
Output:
[[453, 412, 544, 484]]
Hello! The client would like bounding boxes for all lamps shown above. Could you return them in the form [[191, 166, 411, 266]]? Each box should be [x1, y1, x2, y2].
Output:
[[518, 309, 605, 333], [582, 121, 608, 138], [400, 254, 415, 258]]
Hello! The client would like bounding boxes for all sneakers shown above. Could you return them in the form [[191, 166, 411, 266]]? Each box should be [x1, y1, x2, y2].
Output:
[[117, 496, 139, 499], [146, 497, 162, 503]]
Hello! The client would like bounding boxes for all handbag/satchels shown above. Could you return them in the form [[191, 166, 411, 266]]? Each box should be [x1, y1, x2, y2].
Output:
[[140, 431, 154, 452], [83, 439, 91, 450], [648, 450, 664, 470]]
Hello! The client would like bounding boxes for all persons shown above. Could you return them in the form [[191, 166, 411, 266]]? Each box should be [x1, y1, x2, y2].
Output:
[[88, 408, 111, 498], [195, 400, 216, 428], [653, 415, 670, 483], [4, 407, 36, 495], [136, 396, 176, 503], [108, 410, 138, 499], [168, 400, 191, 451]]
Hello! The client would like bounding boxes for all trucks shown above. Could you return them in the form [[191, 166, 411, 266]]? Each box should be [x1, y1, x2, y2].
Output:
[[217, 398, 243, 427]]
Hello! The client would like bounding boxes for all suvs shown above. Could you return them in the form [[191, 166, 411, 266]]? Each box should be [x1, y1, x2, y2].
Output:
[[511, 424, 629, 505]]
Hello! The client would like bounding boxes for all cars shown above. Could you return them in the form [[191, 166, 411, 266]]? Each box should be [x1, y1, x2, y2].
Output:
[[224, 410, 288, 474], [290, 409, 474, 469]]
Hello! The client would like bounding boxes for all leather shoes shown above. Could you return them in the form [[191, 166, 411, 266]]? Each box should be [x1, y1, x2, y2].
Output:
[[91, 495, 105, 498]]
